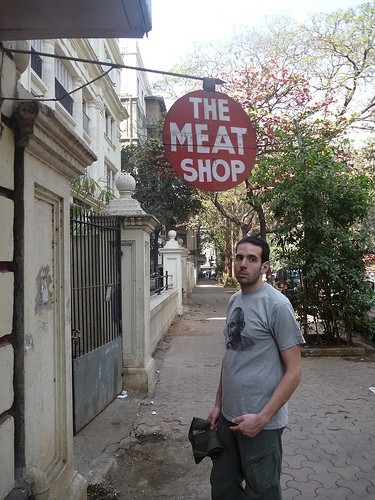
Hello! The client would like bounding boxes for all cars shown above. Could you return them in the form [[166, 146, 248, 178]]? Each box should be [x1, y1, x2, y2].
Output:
[[274, 266, 307, 308]]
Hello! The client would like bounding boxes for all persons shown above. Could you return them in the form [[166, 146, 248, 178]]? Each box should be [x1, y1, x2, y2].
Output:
[[200, 269, 212, 280], [207, 236, 307, 500]]
